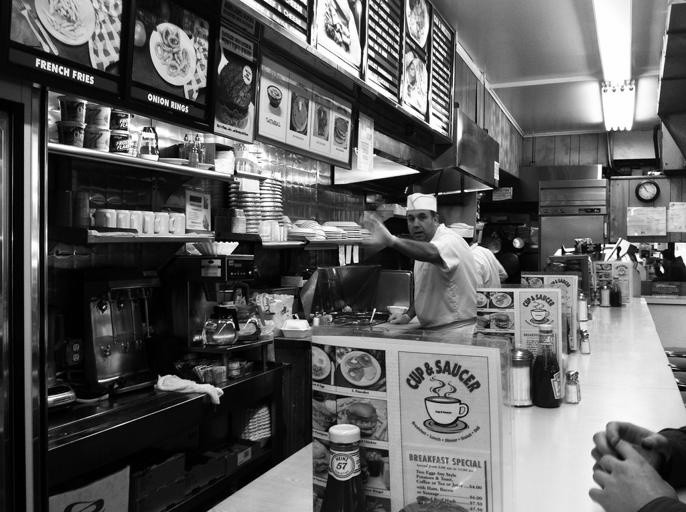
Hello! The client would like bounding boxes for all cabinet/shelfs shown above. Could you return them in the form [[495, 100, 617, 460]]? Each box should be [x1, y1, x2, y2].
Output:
[[236, 138, 387, 247], [48, 139, 235, 248]]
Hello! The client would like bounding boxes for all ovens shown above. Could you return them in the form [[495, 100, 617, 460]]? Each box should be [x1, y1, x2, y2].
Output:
[[537, 181, 606, 272]]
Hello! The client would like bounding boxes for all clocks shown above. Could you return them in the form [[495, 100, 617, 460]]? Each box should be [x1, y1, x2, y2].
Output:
[[635, 180, 660, 203]]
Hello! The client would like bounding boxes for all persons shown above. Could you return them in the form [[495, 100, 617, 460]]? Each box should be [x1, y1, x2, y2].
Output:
[[356, 192, 477, 332], [587, 420, 686, 511], [450, 221, 510, 291]]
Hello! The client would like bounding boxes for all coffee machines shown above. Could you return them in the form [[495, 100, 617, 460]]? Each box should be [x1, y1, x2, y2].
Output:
[[172, 252, 265, 373]]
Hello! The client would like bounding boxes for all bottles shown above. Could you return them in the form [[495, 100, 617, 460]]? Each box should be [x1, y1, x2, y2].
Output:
[[577, 328, 590, 356], [317, 424, 371, 511], [577, 292, 590, 322], [509, 326, 578, 411], [180, 136, 207, 169], [138, 126, 159, 160], [600, 284, 623, 307]]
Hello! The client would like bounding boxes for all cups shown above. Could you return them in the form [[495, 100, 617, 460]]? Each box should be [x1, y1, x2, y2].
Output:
[[531, 309, 548, 321], [423, 396, 469, 424], [265, 85, 283, 106], [95, 208, 187, 236]]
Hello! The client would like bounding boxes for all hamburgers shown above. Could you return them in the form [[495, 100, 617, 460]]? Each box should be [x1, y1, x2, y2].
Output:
[[495, 315, 509, 328], [348, 403, 377, 437]]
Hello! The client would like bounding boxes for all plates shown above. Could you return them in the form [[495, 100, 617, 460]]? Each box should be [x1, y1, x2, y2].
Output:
[[231, 177, 284, 245], [405, 0, 430, 49], [290, 219, 365, 241], [34, 0, 94, 46], [149, 24, 199, 86], [217, 150, 235, 177], [310, 345, 382, 388]]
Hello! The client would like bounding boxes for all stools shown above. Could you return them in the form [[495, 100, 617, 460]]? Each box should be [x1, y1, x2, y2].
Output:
[[664, 346, 686, 405]]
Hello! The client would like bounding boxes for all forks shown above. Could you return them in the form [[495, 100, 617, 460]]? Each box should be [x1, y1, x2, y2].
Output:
[[14, 2, 51, 64]]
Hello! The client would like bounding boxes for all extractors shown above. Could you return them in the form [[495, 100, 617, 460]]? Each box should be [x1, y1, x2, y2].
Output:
[[330, 114, 499, 203]]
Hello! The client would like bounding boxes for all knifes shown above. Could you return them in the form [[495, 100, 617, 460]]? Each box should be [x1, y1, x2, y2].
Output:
[[23, 0, 60, 58]]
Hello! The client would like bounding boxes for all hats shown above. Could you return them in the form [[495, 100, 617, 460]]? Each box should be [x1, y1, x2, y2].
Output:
[[407, 193, 437, 212], [448, 222, 474, 238]]
